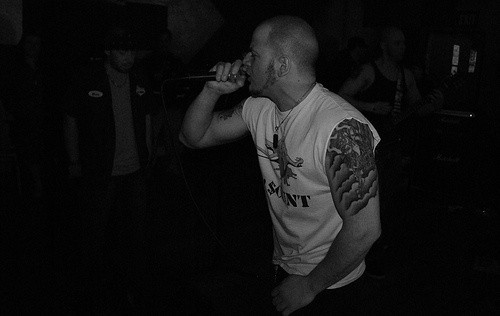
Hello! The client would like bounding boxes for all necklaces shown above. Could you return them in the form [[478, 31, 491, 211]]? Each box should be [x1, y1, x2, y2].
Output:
[[272, 80, 317, 149]]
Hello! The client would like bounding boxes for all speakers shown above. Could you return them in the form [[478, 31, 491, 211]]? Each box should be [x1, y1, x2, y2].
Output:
[[409, 108, 479, 205]]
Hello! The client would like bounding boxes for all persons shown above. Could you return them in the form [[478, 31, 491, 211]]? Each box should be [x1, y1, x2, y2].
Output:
[[2, 30, 228, 300], [181, 15, 384, 316], [338, 24, 444, 211]]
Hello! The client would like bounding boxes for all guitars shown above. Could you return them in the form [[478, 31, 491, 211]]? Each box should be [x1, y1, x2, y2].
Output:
[[369, 84, 437, 152]]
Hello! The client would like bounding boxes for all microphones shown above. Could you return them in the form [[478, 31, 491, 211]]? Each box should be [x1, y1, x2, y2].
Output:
[[169, 69, 241, 83]]
